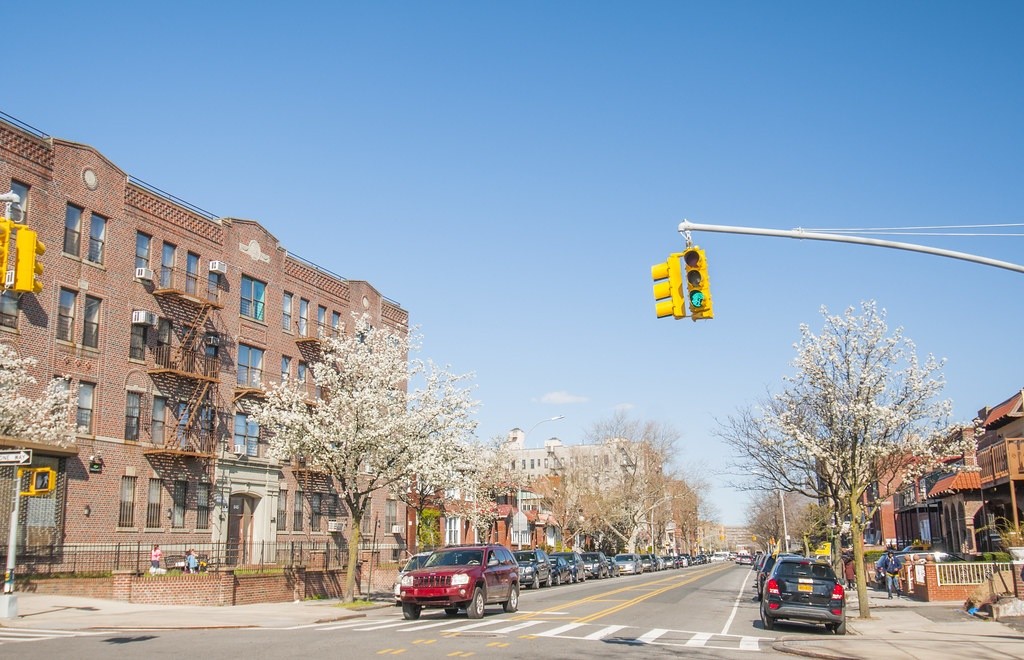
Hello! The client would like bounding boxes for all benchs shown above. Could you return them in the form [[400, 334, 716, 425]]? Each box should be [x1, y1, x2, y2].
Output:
[[163, 554, 216, 571]]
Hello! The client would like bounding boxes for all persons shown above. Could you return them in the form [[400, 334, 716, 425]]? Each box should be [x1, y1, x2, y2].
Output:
[[884, 551, 903, 599], [185, 549, 208, 573], [151, 544, 163, 568], [845, 559, 856, 590]]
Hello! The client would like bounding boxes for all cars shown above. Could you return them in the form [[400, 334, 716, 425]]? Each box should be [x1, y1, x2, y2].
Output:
[[874, 551, 967, 592], [394, 551, 432, 607], [760, 558, 846, 635], [615, 554, 644, 575], [640, 554, 654, 572], [729, 554, 816, 602], [651, 551, 737, 573], [606, 557, 620, 577], [549, 556, 572, 585]]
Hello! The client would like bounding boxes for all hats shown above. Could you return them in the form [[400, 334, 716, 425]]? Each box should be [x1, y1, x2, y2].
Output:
[[888, 550, 894, 556]]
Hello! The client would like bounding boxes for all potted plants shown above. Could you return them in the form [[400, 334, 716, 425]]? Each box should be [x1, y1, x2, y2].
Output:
[[973, 516, 1024, 563]]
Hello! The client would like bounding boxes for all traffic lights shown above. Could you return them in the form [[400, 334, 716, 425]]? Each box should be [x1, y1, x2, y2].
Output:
[[7, 227, 47, 294], [32, 470, 56, 493], [684, 249, 714, 320], [651, 256, 686, 320]]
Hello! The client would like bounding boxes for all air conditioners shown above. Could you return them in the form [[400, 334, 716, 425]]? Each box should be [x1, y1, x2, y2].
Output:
[[132, 310, 157, 326], [206, 336, 220, 347], [210, 260, 227, 275], [233, 444, 248, 455], [328, 522, 344, 533], [136, 268, 153, 280], [391, 525, 405, 534], [5, 269, 14, 286]]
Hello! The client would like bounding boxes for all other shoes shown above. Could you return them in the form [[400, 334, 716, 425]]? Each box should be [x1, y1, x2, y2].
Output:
[[889, 596, 892, 600], [897, 590, 903, 595]]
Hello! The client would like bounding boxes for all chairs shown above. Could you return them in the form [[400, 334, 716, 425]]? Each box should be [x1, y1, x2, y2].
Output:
[[461, 552, 474, 564]]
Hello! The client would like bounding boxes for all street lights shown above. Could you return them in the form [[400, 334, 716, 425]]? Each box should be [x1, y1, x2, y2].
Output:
[[518, 415, 565, 550]]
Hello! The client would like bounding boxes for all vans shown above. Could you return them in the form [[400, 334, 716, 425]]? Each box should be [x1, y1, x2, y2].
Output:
[[902, 545, 932, 551]]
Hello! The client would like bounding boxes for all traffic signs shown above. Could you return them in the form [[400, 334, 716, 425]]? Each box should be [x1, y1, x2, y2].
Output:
[[0, 450, 32, 465]]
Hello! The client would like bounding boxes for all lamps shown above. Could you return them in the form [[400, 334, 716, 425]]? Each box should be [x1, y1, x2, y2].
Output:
[[344, 517, 348, 526], [378, 520, 382, 527], [166, 508, 173, 517], [83, 505, 91, 515], [307, 517, 312, 526]]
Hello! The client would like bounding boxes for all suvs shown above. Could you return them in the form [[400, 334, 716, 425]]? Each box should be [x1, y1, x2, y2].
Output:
[[580, 552, 609, 579], [550, 552, 585, 582], [514, 549, 552, 589], [400, 543, 520, 620]]
